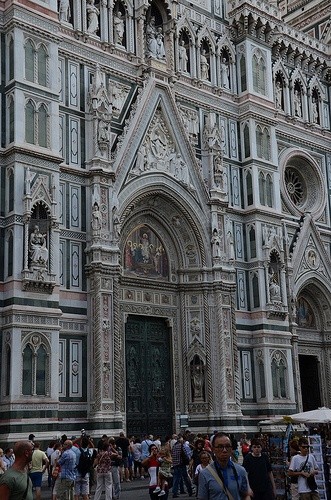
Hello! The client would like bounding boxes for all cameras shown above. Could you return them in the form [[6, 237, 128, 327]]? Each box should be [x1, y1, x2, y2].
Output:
[[81, 428, 86, 437]]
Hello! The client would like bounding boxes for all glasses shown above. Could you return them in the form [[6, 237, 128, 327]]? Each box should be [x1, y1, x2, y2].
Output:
[[213, 445, 233, 450], [301, 445, 309, 449], [151, 448, 157, 450]]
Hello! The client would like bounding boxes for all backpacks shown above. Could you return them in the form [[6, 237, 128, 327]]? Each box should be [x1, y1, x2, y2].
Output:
[[75, 448, 90, 478]]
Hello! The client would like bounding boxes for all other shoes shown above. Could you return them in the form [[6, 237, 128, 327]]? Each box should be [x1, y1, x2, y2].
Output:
[[158, 491, 165, 496], [152, 489, 161, 493]]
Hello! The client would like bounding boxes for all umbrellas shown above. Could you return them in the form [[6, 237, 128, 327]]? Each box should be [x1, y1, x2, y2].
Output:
[[283, 406, 331, 428]]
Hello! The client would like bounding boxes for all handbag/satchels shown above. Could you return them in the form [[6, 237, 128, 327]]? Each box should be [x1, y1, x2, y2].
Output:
[[181, 444, 189, 466], [290, 471, 299, 483], [307, 476, 317, 491]]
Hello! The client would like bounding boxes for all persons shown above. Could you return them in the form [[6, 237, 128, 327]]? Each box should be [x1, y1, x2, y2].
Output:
[[0, 427, 251, 500], [197, 431, 253, 500], [288, 437, 321, 500], [147, 18, 166, 61], [114, 12, 126, 44], [200, 49, 210, 81], [29, 224, 49, 264], [91, 205, 103, 230], [312, 97, 318, 123], [220, 57, 230, 89], [59, 0, 72, 22], [86, 0, 100, 35], [243, 441, 276, 500], [179, 40, 189, 71], [269, 278, 282, 302], [293, 90, 302, 117], [284, 439, 301, 500], [275, 82, 283, 108], [210, 228, 222, 258]]
